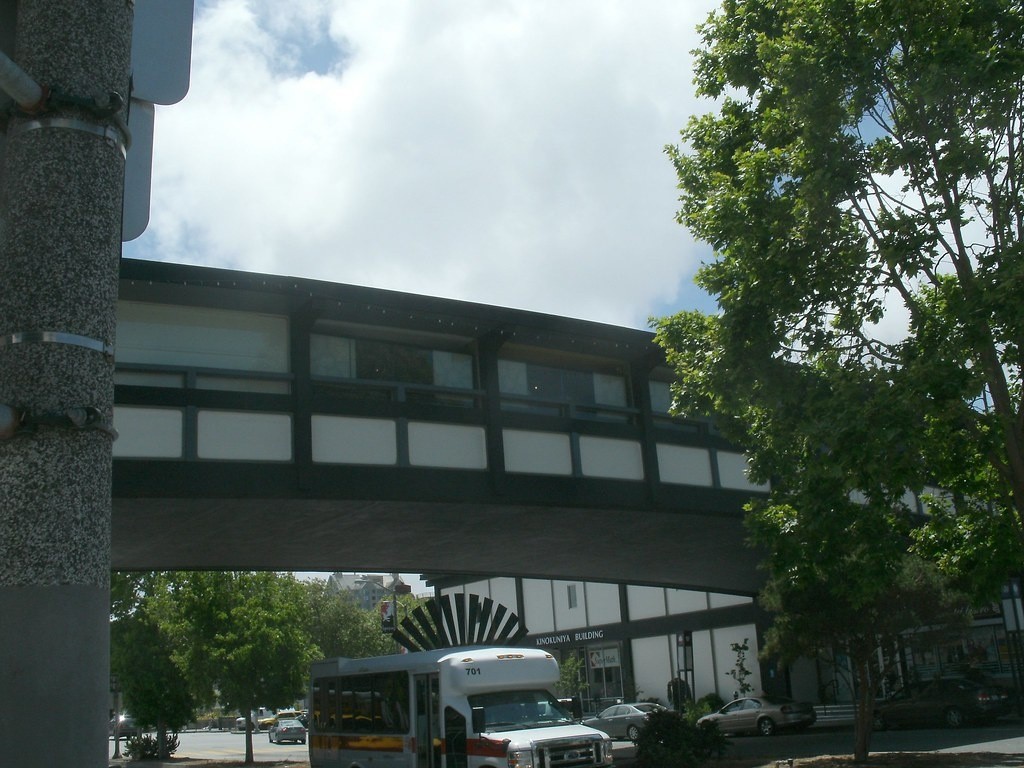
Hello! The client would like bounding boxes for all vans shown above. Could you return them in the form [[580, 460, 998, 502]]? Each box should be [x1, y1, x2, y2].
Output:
[[520, 698, 573, 723]]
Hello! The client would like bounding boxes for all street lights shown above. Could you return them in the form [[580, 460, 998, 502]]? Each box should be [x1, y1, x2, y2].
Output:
[[354, 578, 400, 654]]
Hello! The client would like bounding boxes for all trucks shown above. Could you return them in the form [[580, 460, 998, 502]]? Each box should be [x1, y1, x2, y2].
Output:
[[309, 645, 617, 768], [235, 707, 295, 731]]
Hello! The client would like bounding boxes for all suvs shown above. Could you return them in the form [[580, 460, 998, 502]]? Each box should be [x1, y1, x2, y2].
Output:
[[258, 711, 302, 730]]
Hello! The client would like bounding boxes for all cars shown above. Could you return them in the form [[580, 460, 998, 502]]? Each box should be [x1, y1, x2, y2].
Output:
[[109, 714, 139, 740], [269, 720, 306, 744], [862, 676, 1017, 732], [583, 702, 669, 742], [295, 712, 309, 728], [696, 695, 817, 737]]
